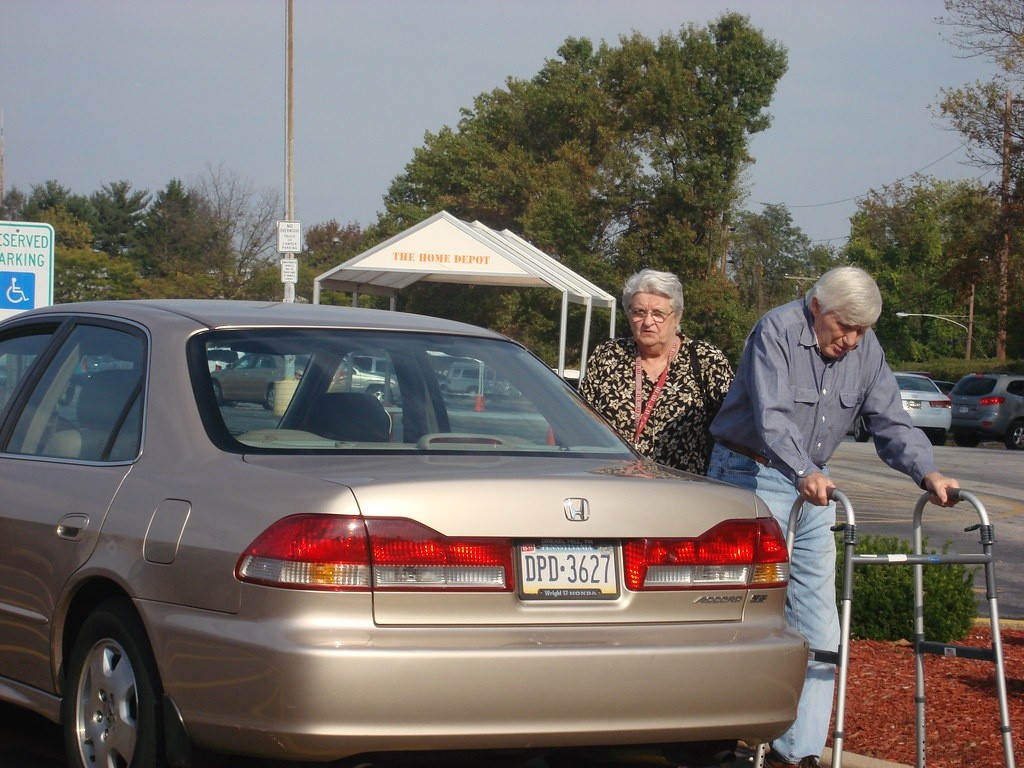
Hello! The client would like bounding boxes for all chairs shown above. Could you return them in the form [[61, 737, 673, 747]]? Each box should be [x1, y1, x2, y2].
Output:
[[39, 364, 145, 463], [301, 390, 390, 445]]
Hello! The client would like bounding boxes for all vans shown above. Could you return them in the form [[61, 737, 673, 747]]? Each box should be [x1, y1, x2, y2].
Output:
[[445, 360, 513, 398]]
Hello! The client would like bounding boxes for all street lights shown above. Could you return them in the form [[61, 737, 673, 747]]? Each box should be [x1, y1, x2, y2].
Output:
[[897, 312, 972, 360]]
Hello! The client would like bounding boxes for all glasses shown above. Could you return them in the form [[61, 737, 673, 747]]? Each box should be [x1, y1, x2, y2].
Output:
[[626, 308, 673, 323]]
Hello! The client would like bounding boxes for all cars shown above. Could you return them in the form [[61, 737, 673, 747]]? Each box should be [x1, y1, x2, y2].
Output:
[[355, 355, 389, 375], [948, 371, 1024, 451], [345, 357, 402, 407], [206, 347, 248, 373], [933, 380, 957, 395], [0, 298, 809, 768], [846, 372, 952, 445], [552, 369, 581, 390], [210, 351, 352, 410]]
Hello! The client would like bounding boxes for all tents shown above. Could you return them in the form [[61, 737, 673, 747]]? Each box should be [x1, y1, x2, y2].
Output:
[[312, 210, 617, 392]]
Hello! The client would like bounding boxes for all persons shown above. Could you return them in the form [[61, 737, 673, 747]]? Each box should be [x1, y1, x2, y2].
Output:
[[577, 268, 739, 477], [705, 266, 960, 766]]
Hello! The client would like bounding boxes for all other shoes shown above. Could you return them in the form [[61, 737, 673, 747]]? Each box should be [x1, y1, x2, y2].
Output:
[[765, 748, 819, 768], [736, 740, 771, 757]]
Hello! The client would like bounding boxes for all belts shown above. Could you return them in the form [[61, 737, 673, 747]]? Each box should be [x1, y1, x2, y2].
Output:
[[718, 438, 770, 466]]
